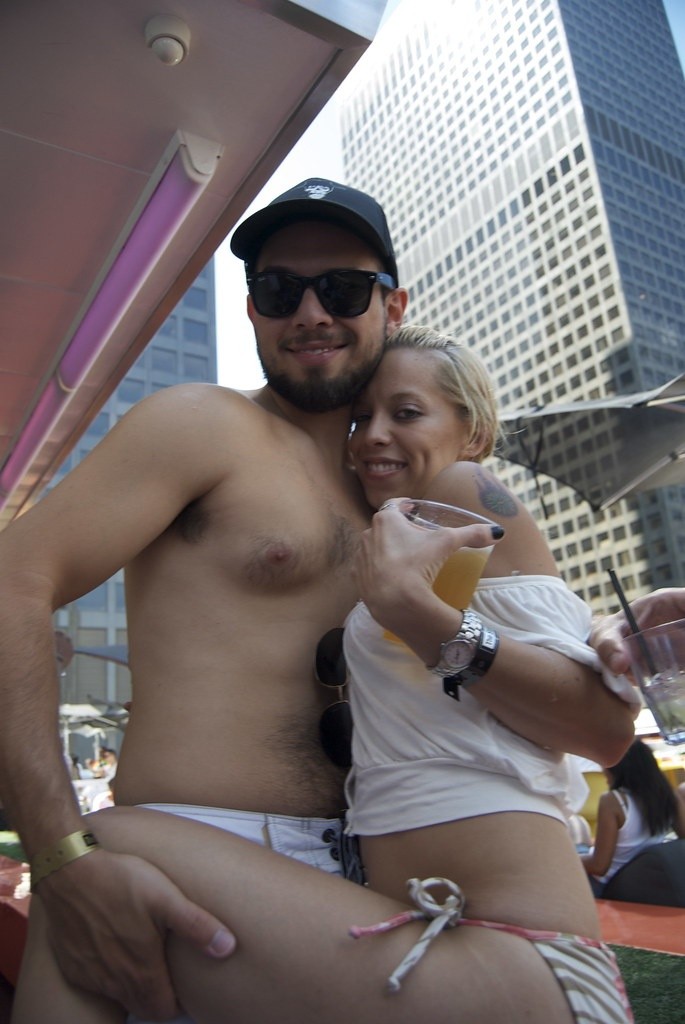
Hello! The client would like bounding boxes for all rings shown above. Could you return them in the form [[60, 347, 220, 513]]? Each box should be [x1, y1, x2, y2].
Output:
[[379, 504, 397, 511]]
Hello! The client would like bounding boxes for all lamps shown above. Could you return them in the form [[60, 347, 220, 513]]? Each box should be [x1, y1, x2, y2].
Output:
[[0, 129, 236, 514]]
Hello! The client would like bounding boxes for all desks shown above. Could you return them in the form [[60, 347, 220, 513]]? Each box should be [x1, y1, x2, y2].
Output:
[[657, 756, 685, 791], [0, 853, 33, 988]]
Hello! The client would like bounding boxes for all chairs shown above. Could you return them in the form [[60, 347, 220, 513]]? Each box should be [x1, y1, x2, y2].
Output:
[[578, 771, 609, 838]]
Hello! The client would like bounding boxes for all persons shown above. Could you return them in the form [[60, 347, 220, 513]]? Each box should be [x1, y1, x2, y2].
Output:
[[73, 747, 118, 811], [0, 177, 684, 1024], [579, 739, 685, 898], [11, 327, 635, 1024]]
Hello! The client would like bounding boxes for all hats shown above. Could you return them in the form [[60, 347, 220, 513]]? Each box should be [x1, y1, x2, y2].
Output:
[[230, 178, 398, 288]]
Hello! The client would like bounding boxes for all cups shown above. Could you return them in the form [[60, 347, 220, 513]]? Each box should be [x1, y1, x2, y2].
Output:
[[621, 617, 685, 748], [382, 499, 500, 649]]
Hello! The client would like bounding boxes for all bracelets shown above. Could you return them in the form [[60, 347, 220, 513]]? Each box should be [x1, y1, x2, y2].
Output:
[[443, 625, 499, 702], [30, 829, 103, 892]]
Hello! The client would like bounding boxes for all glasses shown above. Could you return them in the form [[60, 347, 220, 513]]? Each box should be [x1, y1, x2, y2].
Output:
[[247, 269, 396, 318], [315, 625, 354, 769]]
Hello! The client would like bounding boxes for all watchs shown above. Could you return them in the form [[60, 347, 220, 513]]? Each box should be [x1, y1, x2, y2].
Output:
[[427, 610, 484, 679]]
